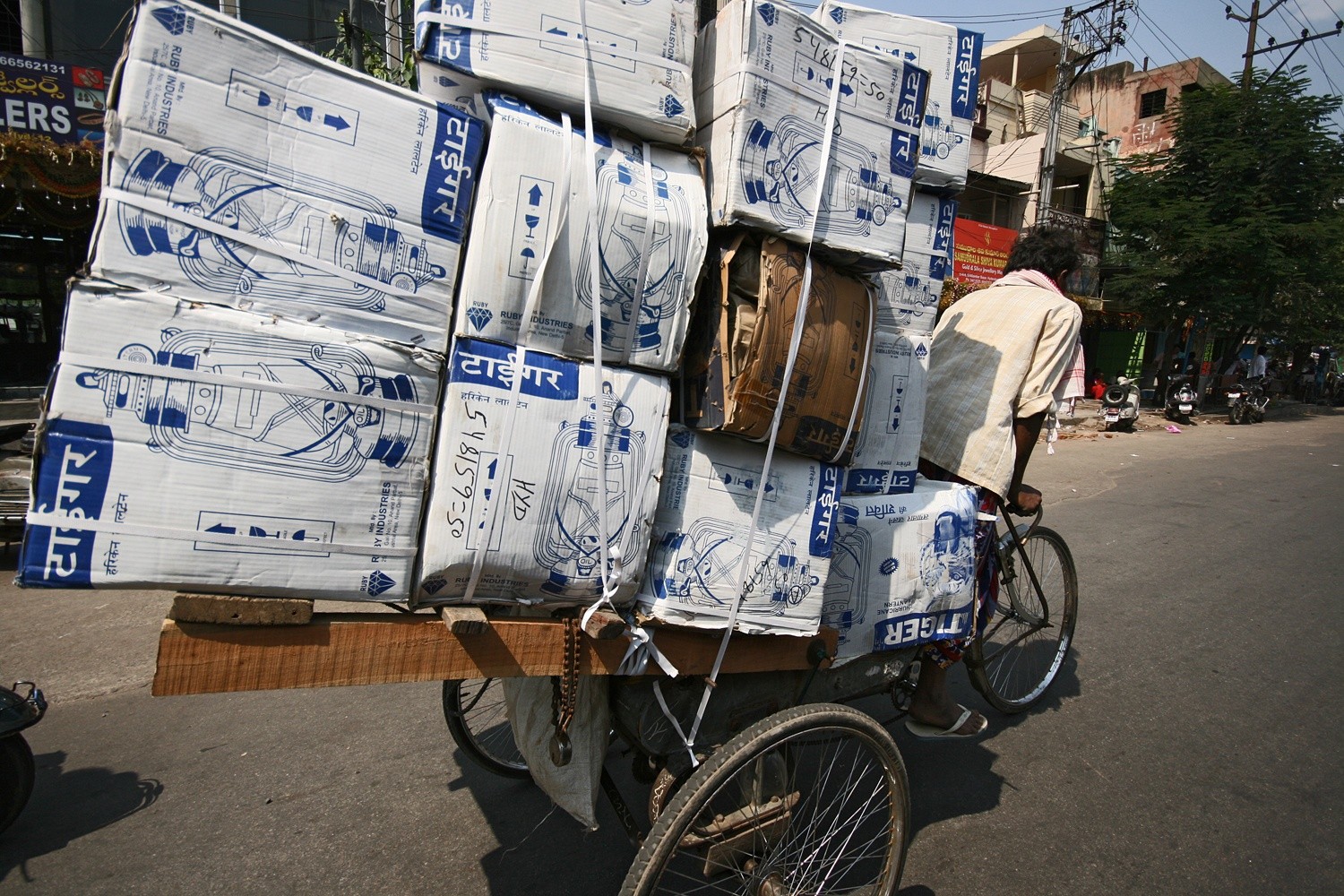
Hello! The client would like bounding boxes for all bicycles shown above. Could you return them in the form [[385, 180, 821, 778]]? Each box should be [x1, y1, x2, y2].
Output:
[[1302, 380, 1314, 404]]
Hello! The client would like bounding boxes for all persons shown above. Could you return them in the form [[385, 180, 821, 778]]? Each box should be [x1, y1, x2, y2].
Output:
[[1150, 336, 1319, 408], [902, 222, 1084, 744]]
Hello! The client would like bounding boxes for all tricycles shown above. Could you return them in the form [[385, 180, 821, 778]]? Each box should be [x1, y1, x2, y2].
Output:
[[446, 493, 1079, 895]]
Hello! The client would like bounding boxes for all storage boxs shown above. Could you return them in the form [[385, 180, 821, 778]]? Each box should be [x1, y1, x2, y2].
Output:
[[9, 1, 978, 690]]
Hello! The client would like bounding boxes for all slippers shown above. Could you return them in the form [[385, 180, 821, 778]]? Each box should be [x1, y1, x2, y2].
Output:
[[904, 704, 989, 740]]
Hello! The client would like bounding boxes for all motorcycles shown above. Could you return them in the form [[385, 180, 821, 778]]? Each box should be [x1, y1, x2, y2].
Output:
[[1224, 374, 1274, 424], [1164, 366, 1199, 423], [1102, 376, 1146, 432], [0, 678, 46, 825]]
